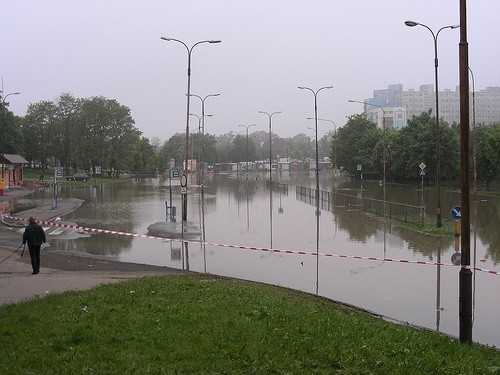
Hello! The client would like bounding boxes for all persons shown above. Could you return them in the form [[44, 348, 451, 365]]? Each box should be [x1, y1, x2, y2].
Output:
[[39, 171, 43, 182], [23, 215, 46, 275]]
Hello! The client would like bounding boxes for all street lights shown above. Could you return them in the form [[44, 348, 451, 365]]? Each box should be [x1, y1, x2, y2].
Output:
[[258, 110, 283, 180], [348, 99, 386, 217], [184, 93, 220, 197], [189, 113, 213, 184], [404, 19, 460, 228], [306, 117, 337, 174], [298, 85, 333, 190], [160, 34, 222, 221], [238, 124, 257, 179], [230, 130, 245, 177]]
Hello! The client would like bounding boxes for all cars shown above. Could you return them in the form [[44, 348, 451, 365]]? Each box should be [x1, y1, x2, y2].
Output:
[[66, 172, 89, 182]]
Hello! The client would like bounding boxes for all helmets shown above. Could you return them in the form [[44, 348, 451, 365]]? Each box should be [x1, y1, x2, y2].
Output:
[[28, 216, 37, 223]]
